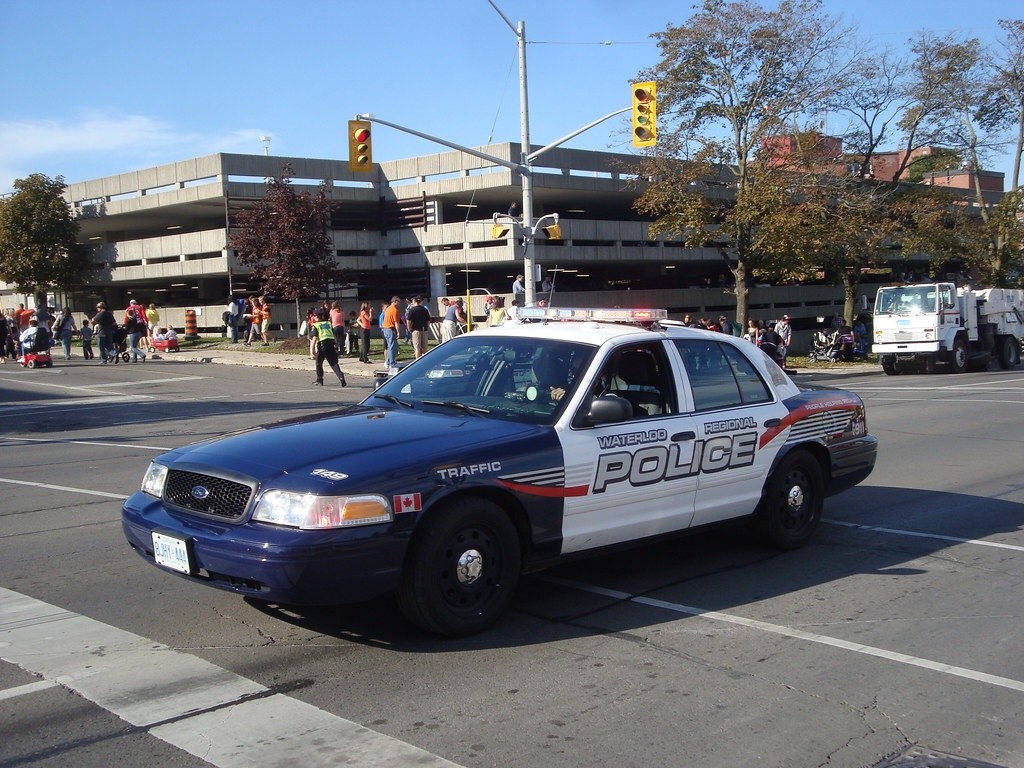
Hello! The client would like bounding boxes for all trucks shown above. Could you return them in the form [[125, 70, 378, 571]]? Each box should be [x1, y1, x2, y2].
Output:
[[862, 282, 1024, 377]]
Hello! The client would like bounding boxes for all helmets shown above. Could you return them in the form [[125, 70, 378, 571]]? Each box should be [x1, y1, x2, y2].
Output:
[[781, 314, 792, 321]]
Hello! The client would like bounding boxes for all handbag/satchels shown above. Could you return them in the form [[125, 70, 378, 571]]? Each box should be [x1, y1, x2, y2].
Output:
[[132, 308, 143, 324], [52, 329, 60, 340], [299, 321, 308, 336], [126, 319, 138, 333]]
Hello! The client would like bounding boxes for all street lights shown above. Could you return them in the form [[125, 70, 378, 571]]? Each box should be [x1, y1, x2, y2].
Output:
[[261, 135, 271, 183]]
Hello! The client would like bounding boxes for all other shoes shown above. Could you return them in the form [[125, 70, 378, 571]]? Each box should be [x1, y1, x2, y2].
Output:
[[141, 355, 147, 362], [85, 356, 89, 360], [90, 354, 93, 359], [359, 359, 364, 362], [244, 343, 251, 347], [364, 361, 372, 364], [262, 342, 270, 346], [312, 381, 324, 386], [131, 360, 136, 363], [341, 382, 346, 386], [99, 360, 107, 364]]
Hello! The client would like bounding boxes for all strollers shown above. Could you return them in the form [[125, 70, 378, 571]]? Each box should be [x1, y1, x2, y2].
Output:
[[807, 330, 854, 363], [99, 323, 130, 364]]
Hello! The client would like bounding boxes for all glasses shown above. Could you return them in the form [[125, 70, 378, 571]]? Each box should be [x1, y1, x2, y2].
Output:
[[395, 300, 401, 303]]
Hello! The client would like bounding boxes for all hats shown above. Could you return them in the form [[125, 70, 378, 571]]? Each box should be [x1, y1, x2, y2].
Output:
[[96, 302, 105, 308], [456, 301, 463, 307]]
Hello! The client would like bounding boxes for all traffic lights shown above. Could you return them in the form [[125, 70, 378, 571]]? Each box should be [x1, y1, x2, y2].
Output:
[[631, 80, 657, 148], [491, 224, 510, 239], [541, 224, 561, 240], [347, 119, 373, 173]]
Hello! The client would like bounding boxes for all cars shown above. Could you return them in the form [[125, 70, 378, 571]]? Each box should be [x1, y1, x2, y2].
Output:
[[120, 304, 880, 639]]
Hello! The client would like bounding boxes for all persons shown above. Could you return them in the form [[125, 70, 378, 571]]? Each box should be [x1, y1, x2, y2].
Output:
[[684, 312, 792, 368], [306, 300, 346, 387], [512, 275, 525, 301], [0, 303, 56, 364], [541, 273, 556, 292], [484, 295, 520, 327], [226, 295, 272, 347], [549, 348, 615, 424], [356, 303, 376, 364], [51, 306, 79, 360], [139, 302, 177, 352], [508, 201, 523, 218], [79, 319, 94, 360], [530, 295, 547, 323], [90, 301, 119, 365], [813, 311, 868, 360], [378, 296, 432, 368], [441, 298, 466, 343], [122, 298, 149, 364], [348, 310, 360, 356]]
